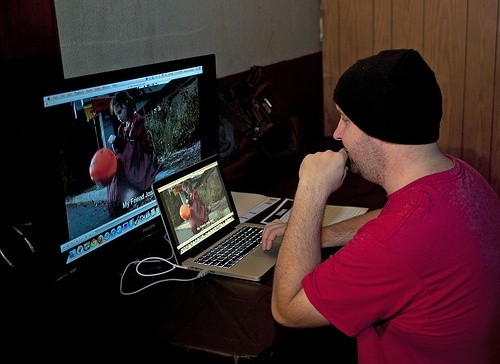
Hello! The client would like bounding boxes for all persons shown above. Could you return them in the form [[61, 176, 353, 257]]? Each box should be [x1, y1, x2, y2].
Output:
[[181, 181, 209, 233], [106, 91, 163, 219], [260, 46, 500, 364]]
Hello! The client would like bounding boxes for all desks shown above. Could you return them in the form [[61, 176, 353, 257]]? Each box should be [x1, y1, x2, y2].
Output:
[[154, 270, 279, 364]]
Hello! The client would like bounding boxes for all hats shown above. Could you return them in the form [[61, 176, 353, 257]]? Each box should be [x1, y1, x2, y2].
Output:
[[333, 49, 443, 144]]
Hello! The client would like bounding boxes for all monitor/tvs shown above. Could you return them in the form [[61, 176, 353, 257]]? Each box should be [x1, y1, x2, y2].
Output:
[[36, 54, 220, 282]]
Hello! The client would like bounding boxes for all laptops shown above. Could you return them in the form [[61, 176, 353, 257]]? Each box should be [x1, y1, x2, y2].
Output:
[[151, 153, 284, 281]]
